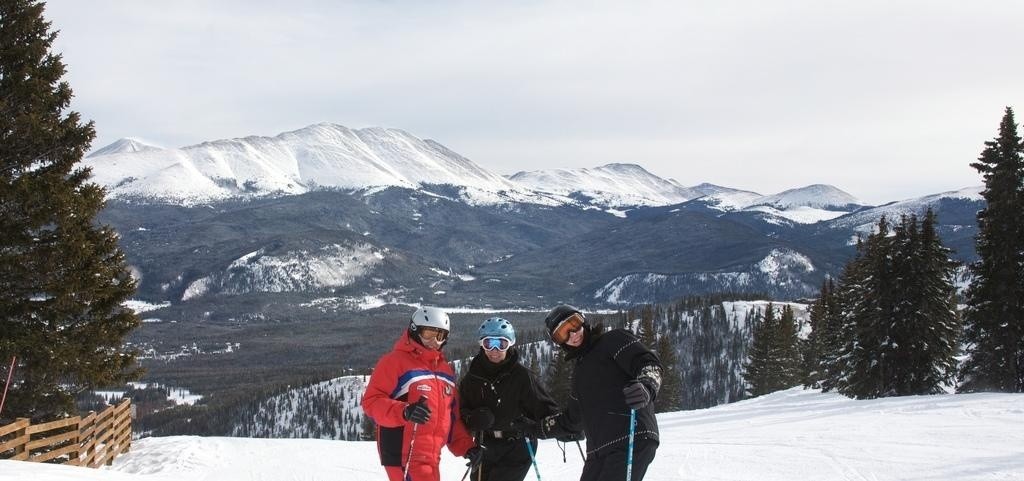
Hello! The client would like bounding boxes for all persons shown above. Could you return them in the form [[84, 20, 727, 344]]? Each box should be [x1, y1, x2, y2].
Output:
[[458, 314, 561, 480], [508, 303, 666, 481], [357, 303, 483, 481]]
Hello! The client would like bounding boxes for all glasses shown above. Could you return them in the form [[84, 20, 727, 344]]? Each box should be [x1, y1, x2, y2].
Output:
[[478, 337, 516, 352], [550, 312, 585, 345], [410, 315, 449, 342]]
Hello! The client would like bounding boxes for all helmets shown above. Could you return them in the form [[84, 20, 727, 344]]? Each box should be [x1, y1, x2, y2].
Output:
[[408, 306, 450, 351], [545, 305, 582, 333], [479, 317, 515, 342]]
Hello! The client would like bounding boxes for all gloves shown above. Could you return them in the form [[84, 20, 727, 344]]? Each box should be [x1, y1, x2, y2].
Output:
[[466, 408, 495, 431], [508, 416, 537, 438], [402, 402, 432, 424], [556, 430, 585, 442], [623, 382, 650, 411]]
